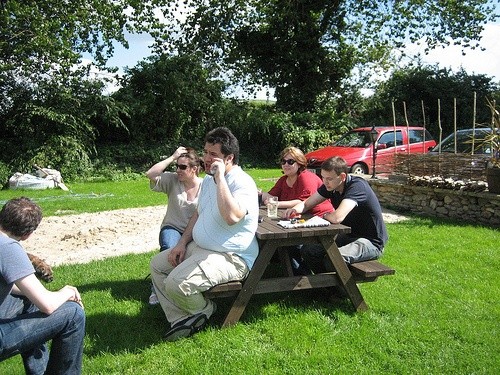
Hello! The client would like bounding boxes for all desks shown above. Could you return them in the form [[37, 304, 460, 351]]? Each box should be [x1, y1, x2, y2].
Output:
[[220, 203, 370, 329]]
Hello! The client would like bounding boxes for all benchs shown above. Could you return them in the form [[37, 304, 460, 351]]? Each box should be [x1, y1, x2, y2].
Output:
[[347, 259, 395, 284], [204, 281, 241, 303]]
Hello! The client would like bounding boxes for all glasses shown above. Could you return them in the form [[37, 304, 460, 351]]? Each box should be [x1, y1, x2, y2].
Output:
[[281, 159, 298, 165], [175, 163, 194, 170]]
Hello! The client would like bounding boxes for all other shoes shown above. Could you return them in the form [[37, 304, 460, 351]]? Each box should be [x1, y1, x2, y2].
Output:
[[150, 294, 160, 305]]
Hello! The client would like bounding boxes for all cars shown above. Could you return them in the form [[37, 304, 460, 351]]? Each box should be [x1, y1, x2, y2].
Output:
[[430, 128, 500, 156]]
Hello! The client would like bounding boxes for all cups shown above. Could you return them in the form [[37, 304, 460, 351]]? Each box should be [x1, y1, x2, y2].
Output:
[[268, 196, 278, 217]]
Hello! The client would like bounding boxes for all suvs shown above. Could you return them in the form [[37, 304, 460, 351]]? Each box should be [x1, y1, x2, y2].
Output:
[[301, 126, 438, 178]]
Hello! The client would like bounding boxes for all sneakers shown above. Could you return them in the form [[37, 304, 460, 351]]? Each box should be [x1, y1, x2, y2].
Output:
[[163, 314, 208, 342], [207, 302, 227, 328]]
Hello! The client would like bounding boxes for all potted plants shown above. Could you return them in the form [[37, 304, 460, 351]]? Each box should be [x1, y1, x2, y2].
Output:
[[463, 95, 500, 192]]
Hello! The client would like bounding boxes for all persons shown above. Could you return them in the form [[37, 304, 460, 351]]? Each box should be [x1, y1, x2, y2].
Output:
[[0, 197, 86, 375], [146, 146, 206, 306], [261, 146, 335, 271], [282, 156, 388, 305], [149, 127, 259, 343]]
